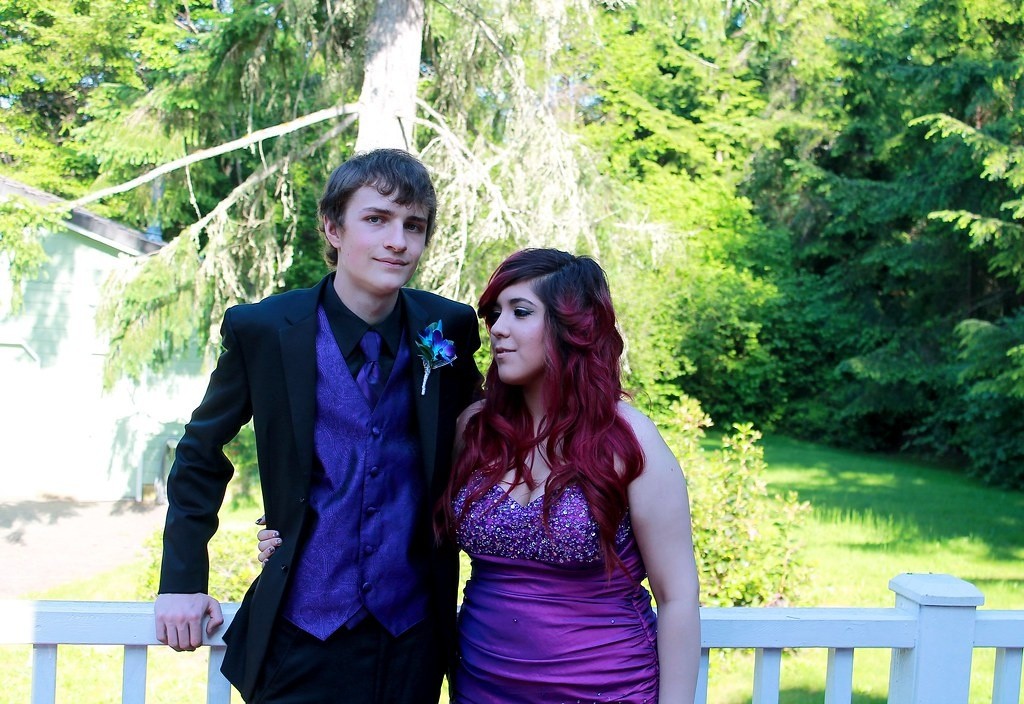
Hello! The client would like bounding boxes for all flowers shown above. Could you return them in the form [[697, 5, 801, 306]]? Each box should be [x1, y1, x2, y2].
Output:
[[415, 320, 457, 395]]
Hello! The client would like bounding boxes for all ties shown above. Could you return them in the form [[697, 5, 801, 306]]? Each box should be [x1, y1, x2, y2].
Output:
[[356, 331, 385, 411]]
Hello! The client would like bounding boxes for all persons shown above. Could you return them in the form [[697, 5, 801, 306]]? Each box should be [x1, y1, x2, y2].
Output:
[[255, 247, 700, 704], [153, 148, 485, 704]]
